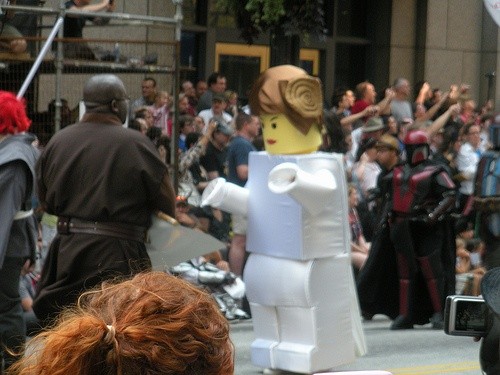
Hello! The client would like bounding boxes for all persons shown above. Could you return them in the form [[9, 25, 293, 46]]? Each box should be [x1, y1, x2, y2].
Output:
[[0, 90, 43, 371], [32, 74, 177, 323], [354, 130, 459, 332], [3, 270, 237, 375], [10, 70, 500, 321], [474, 265, 500, 375]]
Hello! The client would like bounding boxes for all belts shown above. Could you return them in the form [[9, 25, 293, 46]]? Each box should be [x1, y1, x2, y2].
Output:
[[57, 216, 148, 242]]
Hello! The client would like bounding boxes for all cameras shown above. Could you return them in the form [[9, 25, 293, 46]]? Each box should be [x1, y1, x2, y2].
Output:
[[443, 295, 488, 337]]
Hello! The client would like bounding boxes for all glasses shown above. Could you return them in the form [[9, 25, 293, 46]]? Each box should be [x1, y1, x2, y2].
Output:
[[109, 95, 131, 104]]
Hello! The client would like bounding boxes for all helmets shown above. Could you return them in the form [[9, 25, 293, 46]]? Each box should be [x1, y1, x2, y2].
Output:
[[403, 130, 430, 163]]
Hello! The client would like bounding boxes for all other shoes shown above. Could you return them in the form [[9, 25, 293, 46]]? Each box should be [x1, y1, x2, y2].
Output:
[[432, 315, 446, 328], [391, 314, 414, 328]]
